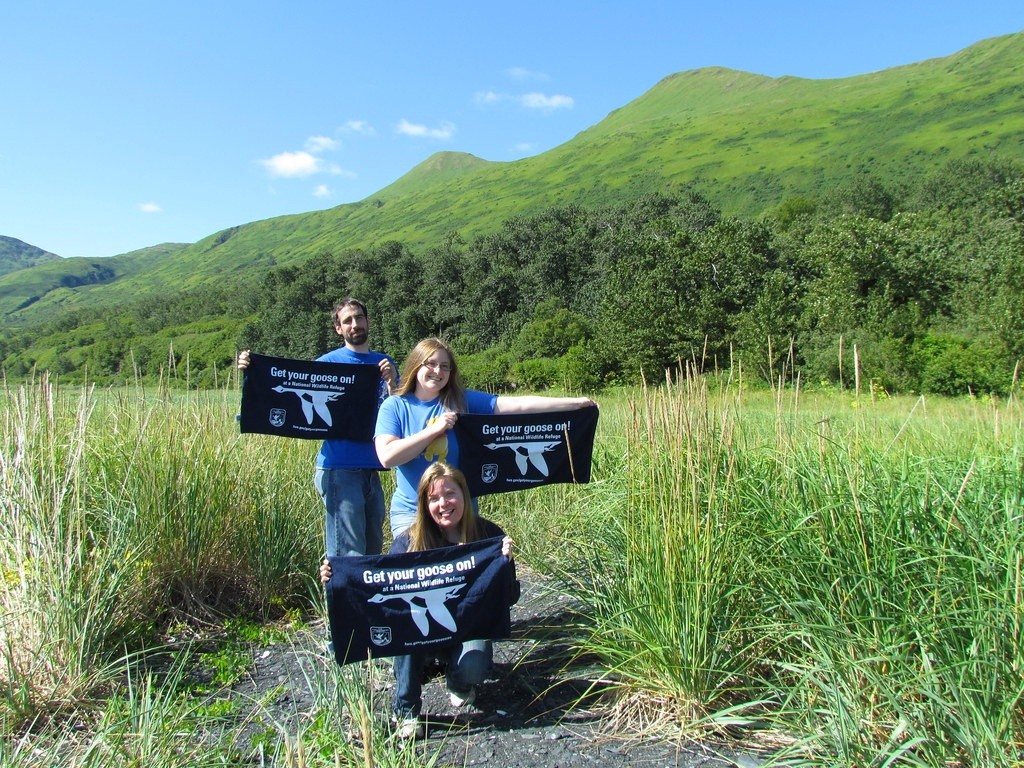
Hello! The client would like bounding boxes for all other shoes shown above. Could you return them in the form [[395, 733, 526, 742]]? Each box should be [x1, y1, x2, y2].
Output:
[[392, 712, 423, 739], [450, 685, 475, 706]]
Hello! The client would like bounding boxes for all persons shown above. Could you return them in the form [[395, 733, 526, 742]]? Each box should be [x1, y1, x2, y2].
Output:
[[372, 337, 601, 686], [318, 461, 517, 741], [240, 296, 401, 655]]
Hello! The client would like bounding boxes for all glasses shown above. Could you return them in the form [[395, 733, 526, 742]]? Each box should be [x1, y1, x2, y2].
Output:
[[422, 360, 453, 371]]
